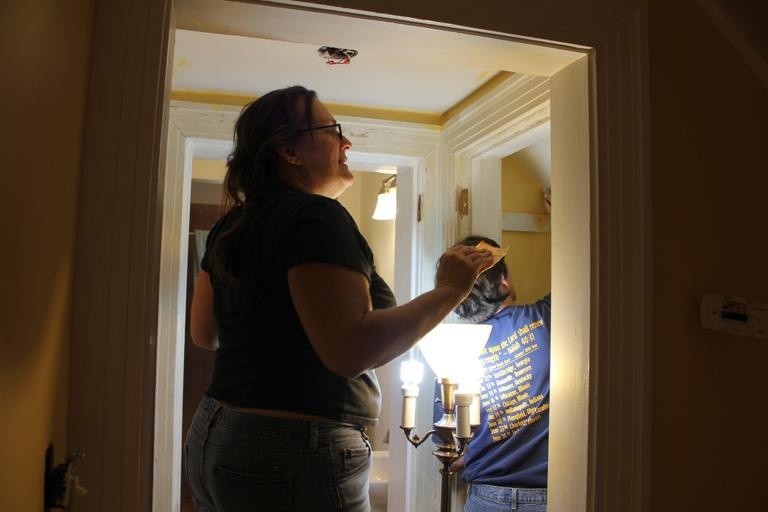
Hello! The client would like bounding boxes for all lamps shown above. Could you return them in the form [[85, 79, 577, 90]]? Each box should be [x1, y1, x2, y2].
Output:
[[398, 320, 493, 511], [371, 173, 397, 222]]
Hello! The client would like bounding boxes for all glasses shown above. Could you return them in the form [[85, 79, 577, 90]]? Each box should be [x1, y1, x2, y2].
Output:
[[308, 120, 344, 140]]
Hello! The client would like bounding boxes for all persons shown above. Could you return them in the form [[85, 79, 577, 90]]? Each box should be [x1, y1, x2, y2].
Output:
[[431, 187, 551, 512], [182, 84, 494, 512]]
